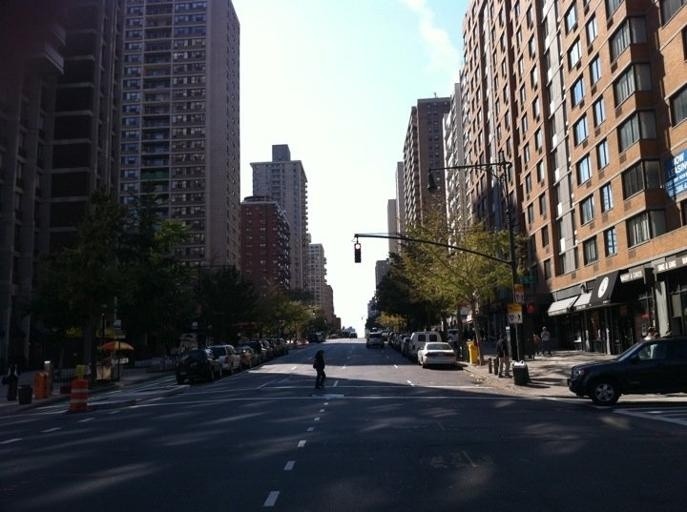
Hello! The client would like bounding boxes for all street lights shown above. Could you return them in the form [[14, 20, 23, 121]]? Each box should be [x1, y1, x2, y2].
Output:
[[428, 161, 525, 362]]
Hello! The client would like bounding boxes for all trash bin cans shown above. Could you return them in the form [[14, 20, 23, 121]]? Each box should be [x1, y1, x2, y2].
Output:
[[16, 384, 33, 404], [513, 364, 529, 385]]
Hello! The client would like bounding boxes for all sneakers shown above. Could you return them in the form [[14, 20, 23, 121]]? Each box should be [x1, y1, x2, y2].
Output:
[[499, 373, 512, 378]]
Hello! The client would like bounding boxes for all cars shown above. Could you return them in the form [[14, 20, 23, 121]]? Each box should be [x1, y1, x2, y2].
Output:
[[387, 331, 456, 368], [567, 336, 687, 406], [175, 337, 288, 385], [367, 332, 384, 349]]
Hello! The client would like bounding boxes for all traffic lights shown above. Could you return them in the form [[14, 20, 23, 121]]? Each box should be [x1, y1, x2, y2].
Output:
[[354, 243, 362, 262]]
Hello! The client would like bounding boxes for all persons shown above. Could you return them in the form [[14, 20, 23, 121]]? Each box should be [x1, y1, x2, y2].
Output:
[[532, 331, 541, 355], [540, 326, 552, 356], [642, 326, 662, 356], [494, 332, 512, 378], [313, 350, 326, 389], [526, 332, 534, 358]]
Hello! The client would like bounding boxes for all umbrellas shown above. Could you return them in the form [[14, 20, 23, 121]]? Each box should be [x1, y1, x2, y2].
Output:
[[96, 339, 134, 351]]
